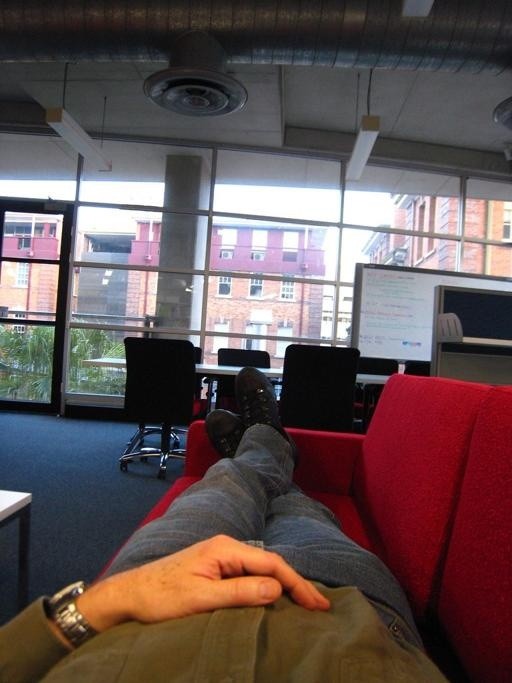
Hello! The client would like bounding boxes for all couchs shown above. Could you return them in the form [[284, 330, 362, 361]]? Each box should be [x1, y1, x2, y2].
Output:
[[76, 374, 512, 683]]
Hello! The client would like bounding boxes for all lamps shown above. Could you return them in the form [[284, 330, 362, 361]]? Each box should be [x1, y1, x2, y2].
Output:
[[345, 68, 381, 181], [44, 62, 114, 173]]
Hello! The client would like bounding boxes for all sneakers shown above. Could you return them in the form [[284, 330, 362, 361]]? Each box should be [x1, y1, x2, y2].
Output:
[[206, 367, 298, 470]]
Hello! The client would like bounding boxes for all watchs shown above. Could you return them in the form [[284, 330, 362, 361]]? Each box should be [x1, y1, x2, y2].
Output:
[[41, 580, 100, 646]]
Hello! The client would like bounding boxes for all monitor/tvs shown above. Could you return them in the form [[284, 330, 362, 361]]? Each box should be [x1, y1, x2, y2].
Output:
[[350, 263, 512, 385]]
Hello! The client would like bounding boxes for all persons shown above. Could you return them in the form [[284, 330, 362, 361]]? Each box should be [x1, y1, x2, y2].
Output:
[[0, 367, 454, 681]]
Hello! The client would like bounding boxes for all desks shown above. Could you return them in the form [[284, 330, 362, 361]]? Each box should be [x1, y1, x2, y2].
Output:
[[85, 357, 391, 433], [0, 489, 32, 574]]
